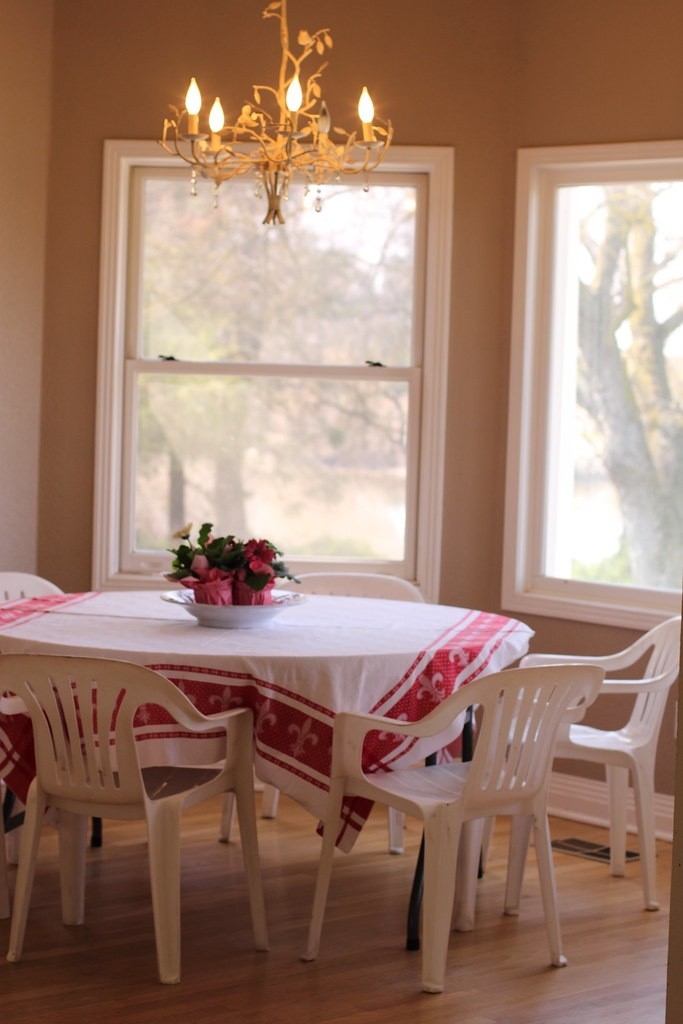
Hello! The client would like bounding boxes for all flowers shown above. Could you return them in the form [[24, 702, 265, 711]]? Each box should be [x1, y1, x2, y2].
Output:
[[237, 540, 300, 588], [163, 522, 238, 581]]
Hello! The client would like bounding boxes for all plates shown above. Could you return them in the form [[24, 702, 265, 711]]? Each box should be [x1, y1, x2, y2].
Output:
[[159, 587, 307, 629]]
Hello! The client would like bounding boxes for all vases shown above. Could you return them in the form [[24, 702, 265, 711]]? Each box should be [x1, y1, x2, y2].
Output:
[[189, 578, 233, 606], [231, 582, 270, 606]]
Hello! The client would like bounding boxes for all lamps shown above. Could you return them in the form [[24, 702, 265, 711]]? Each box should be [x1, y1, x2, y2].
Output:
[[160, 0, 392, 227]]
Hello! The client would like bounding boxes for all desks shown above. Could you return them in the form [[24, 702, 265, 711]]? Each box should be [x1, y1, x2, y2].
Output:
[[0, 590, 533, 933]]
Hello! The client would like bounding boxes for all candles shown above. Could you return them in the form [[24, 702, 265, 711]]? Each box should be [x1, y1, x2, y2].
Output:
[[183, 76, 379, 153]]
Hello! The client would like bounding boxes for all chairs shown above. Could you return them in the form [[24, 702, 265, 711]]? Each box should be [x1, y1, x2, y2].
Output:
[[478, 614, 681, 916], [302, 664, 605, 992], [1, 656, 269, 986], [0, 570, 102, 865]]
